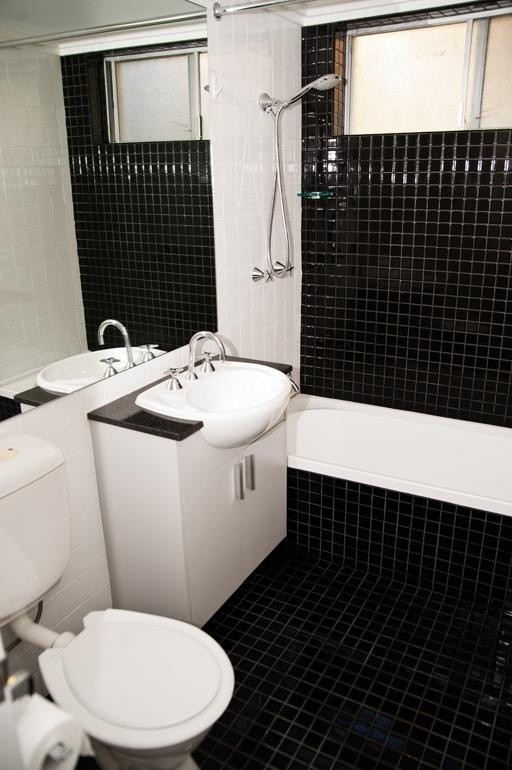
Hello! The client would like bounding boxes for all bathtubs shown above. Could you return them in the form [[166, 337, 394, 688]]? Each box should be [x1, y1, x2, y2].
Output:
[[288, 394, 510, 610]]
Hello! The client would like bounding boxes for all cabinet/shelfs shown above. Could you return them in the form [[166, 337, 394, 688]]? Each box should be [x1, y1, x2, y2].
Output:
[[91, 419, 288, 628]]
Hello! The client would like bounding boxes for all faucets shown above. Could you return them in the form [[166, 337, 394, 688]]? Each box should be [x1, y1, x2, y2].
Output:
[[289, 379, 299, 392], [98, 319, 133, 362], [188, 331, 225, 378]]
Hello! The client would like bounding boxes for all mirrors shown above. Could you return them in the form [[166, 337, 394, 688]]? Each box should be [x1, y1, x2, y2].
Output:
[[0, 0, 218, 422]]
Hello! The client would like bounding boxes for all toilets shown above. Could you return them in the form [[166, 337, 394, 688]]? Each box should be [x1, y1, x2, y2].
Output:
[[0, 435, 237, 769]]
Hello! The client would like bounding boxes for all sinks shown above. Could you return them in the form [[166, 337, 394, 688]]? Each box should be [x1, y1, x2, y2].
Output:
[[135, 361, 292, 448], [37, 347, 167, 393]]
